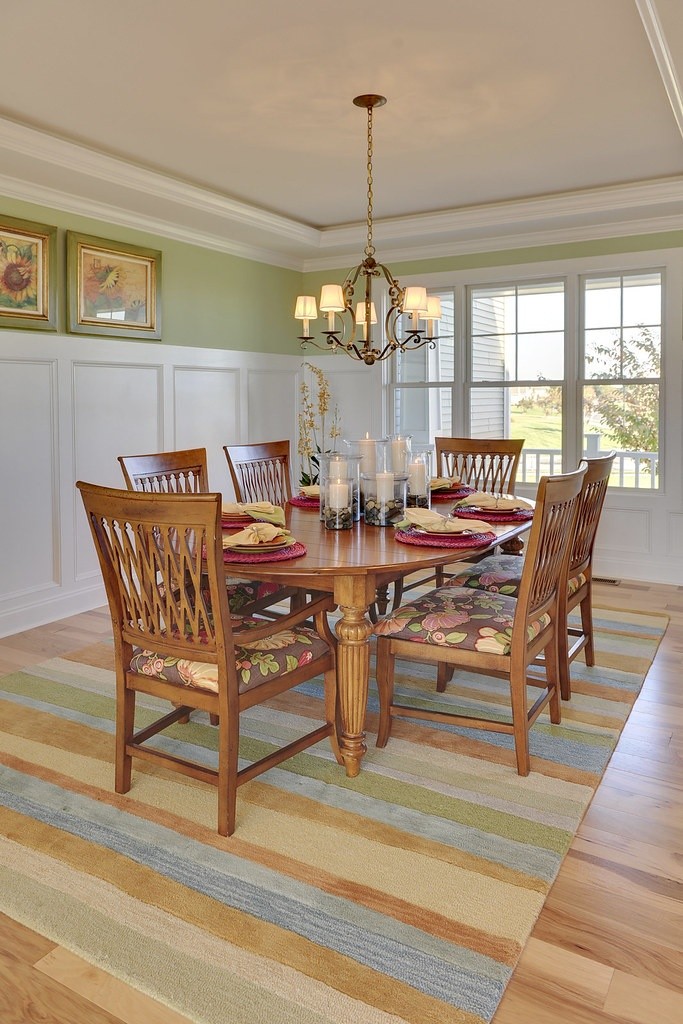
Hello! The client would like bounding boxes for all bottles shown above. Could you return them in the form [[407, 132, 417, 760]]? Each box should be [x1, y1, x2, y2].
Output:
[[314, 432, 432, 529]]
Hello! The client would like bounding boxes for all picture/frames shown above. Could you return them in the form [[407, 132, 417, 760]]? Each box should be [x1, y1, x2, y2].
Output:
[[0, 213, 59, 333], [65, 229, 164, 342]]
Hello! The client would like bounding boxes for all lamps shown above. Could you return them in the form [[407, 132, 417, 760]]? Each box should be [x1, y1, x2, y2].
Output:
[[294, 95, 442, 367]]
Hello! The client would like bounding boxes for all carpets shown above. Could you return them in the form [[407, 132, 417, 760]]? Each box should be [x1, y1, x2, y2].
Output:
[[0, 582, 672, 1024]]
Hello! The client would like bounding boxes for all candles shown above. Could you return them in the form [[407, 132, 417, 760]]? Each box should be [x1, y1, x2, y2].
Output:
[[382, 432, 413, 497], [360, 470, 413, 527], [401, 448, 434, 509], [313, 453, 365, 522], [340, 430, 391, 516], [321, 475, 355, 531]]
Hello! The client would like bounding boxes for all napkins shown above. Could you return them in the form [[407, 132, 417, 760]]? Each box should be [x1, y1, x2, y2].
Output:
[[449, 492, 534, 512], [222, 523, 292, 550], [299, 485, 319, 499], [223, 501, 286, 526], [392, 507, 493, 534], [431, 476, 461, 490]]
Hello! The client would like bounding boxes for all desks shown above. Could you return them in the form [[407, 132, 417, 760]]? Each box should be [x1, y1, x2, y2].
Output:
[[157, 492, 536, 779]]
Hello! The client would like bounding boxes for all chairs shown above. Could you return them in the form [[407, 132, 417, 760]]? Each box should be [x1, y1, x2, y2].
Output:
[[372, 461, 589, 778], [442, 450, 618, 702], [115, 448, 302, 727], [76, 481, 347, 838], [223, 440, 378, 632], [391, 437, 526, 610]]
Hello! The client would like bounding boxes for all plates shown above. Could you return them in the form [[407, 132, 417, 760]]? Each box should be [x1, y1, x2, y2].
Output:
[[470, 496, 530, 514], [220, 512, 254, 521], [434, 481, 470, 493], [225, 530, 296, 555], [411, 516, 492, 537], [299, 489, 321, 500]]
